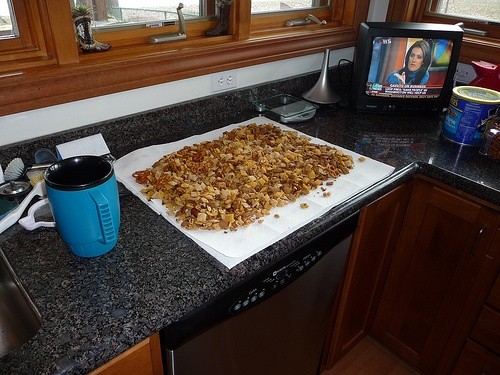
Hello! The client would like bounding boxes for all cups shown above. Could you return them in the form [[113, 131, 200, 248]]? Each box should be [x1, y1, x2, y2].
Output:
[[44, 154, 121, 258], [0, 179, 35, 224]]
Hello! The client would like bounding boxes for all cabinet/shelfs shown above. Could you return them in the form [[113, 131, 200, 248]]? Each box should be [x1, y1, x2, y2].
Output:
[[361, 175, 500, 375], [329, 183, 416, 367]]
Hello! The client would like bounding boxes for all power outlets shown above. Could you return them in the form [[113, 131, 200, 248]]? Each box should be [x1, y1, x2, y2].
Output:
[[210, 71, 237, 93]]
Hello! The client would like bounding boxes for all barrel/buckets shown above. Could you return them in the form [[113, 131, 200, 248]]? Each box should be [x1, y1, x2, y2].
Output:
[[441, 86, 500, 147]]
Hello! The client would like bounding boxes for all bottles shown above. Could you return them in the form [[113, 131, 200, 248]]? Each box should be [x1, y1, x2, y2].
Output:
[[478, 106, 500, 170]]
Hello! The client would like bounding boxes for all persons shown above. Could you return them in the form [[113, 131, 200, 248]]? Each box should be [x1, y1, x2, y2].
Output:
[[386, 40, 432, 86]]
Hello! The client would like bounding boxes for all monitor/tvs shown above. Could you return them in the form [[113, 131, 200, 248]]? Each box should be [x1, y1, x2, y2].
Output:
[[347, 22, 463, 116]]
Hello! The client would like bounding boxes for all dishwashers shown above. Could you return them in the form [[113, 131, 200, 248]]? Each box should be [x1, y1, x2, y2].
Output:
[[162, 212, 358, 375]]
